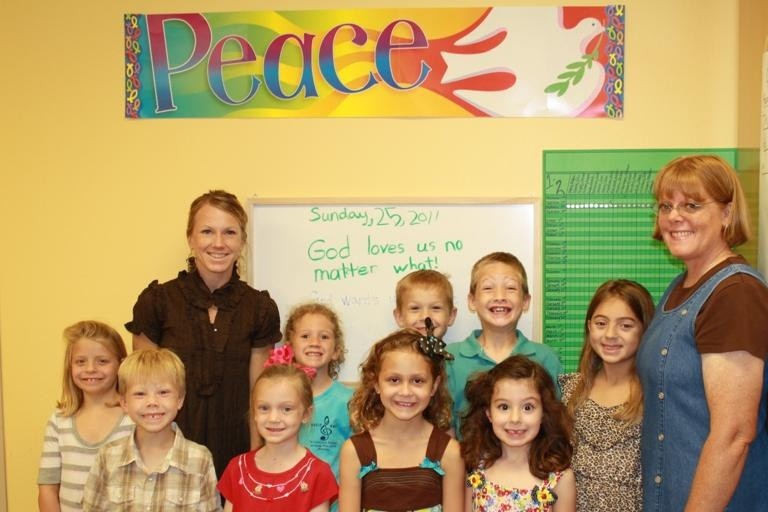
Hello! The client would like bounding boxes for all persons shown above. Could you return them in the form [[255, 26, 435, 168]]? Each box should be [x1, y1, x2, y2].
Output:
[[442, 251, 565, 441], [125, 188, 284, 512], [37, 319, 132, 512], [556, 278, 657, 511], [82, 345, 224, 512], [338, 317, 469, 511], [286, 297, 366, 511], [636, 154, 768, 511], [213, 340, 343, 512], [392, 267, 460, 355], [458, 354, 579, 511]]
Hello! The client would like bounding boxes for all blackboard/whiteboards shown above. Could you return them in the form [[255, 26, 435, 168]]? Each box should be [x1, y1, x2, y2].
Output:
[[240, 198, 543, 394]]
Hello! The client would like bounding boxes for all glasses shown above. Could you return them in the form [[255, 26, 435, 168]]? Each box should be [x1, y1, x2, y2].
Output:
[[656, 201, 716, 213]]
